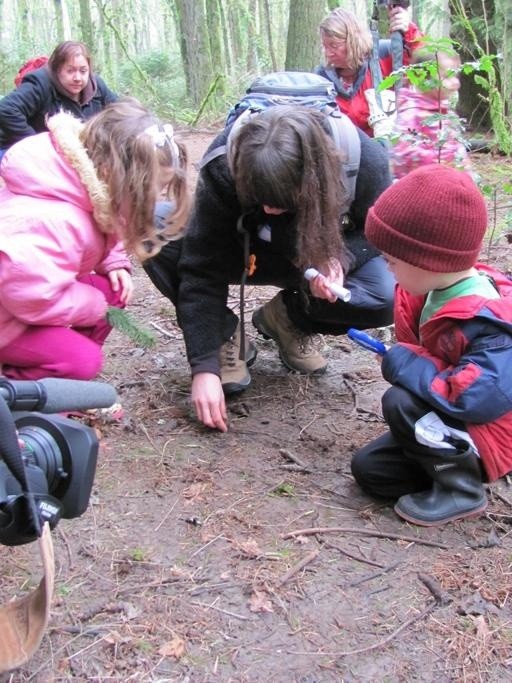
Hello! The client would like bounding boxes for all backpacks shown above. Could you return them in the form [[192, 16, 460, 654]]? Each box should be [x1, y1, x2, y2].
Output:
[[15, 57, 51, 89], [191, 72, 360, 218]]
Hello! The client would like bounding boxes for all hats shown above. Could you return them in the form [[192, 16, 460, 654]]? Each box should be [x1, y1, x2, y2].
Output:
[[363, 165, 488, 272]]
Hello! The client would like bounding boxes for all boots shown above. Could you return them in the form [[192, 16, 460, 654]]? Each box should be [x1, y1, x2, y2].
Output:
[[394, 441, 488, 526]]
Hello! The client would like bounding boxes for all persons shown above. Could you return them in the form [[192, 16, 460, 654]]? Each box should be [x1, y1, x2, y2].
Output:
[[0, 101, 199, 421], [351, 163, 512, 527], [311, 3, 461, 142], [133, 102, 400, 435], [1, 41, 122, 167]]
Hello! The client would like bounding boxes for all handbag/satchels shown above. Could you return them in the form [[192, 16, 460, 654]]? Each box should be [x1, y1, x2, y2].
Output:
[[365, 88, 459, 140]]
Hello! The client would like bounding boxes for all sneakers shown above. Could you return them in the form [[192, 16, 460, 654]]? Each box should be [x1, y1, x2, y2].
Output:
[[218, 319, 258, 394], [58, 404, 123, 422], [250, 290, 328, 377]]
